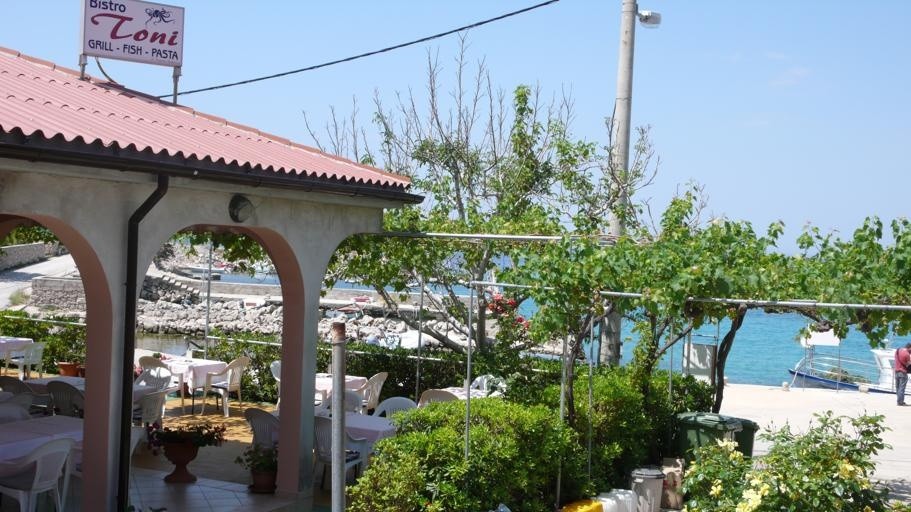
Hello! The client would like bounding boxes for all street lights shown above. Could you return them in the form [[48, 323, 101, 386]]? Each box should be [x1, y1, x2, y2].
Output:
[[599, 0, 662, 369]]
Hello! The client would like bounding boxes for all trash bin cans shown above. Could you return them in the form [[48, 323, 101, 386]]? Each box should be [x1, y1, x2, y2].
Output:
[[629, 467, 666, 512], [676, 412, 759, 472]]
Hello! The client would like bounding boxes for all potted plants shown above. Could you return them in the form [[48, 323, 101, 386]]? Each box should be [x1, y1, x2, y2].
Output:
[[44, 323, 87, 377]]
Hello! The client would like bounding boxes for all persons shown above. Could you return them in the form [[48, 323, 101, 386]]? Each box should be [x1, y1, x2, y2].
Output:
[[894, 343, 911, 406]]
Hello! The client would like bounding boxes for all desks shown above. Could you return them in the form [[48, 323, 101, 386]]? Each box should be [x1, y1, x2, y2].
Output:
[[0, 336, 34, 380]]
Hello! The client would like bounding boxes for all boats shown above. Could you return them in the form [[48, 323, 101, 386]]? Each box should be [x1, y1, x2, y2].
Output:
[[789, 317, 911, 396]]
